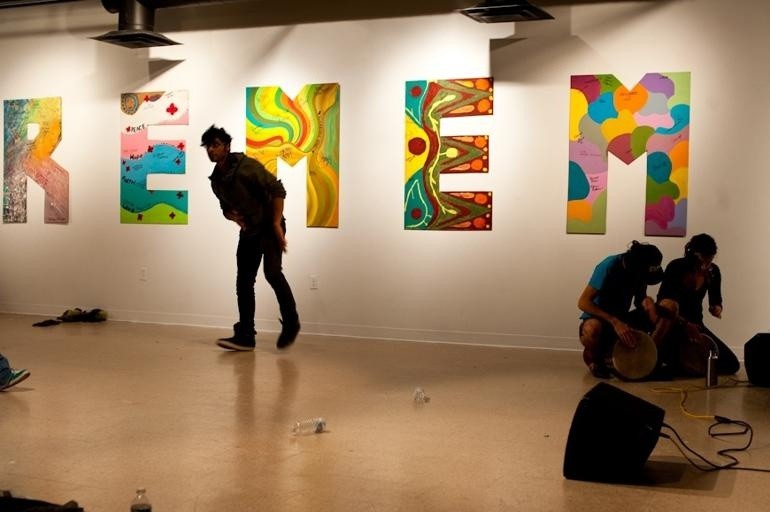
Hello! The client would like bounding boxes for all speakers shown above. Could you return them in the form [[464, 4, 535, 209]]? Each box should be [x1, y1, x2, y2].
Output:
[[562, 382, 665, 485], [743, 333, 770, 388]]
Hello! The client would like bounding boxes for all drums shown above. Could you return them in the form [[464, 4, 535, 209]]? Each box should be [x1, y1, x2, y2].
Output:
[[607, 330, 658, 381]]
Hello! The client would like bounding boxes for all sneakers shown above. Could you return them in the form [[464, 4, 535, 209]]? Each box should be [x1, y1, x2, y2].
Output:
[[216, 333, 255, 352], [277, 318, 300, 348], [0, 368, 31, 391]]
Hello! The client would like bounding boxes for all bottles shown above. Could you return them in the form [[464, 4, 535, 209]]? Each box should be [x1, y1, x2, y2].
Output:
[[286, 417, 326, 434], [705, 348, 719, 388], [128, 488, 152, 512], [413, 385, 425, 403]]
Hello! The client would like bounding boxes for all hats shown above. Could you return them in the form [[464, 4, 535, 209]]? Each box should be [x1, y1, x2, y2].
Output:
[[629, 241, 665, 286]]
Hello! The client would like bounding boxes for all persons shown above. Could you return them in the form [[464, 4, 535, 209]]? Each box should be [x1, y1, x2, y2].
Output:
[[0, 354, 30, 390], [577, 233, 740, 380], [200, 124, 301, 352]]
